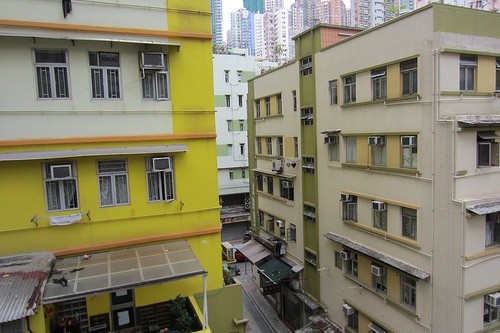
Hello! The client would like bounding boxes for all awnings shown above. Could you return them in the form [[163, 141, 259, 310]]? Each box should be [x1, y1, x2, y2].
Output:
[[254, 256, 292, 284], [234, 239, 272, 265]]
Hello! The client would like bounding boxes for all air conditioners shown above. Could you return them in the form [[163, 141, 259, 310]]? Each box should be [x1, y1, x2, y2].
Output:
[[340, 249, 356, 260], [50, 163, 73, 181], [340, 193, 353, 202], [244, 197, 249, 210], [140, 51, 165, 69], [277, 221, 284, 227], [370, 264, 387, 278], [290, 279, 300, 290], [325, 136, 335, 144], [222, 242, 235, 261], [400, 135, 417, 146], [152, 156, 171, 172], [371, 200, 387, 211], [272, 159, 281, 170], [486, 292, 500, 307], [282, 180, 293, 188], [343, 303, 355, 316], [368, 136, 384, 146]]
